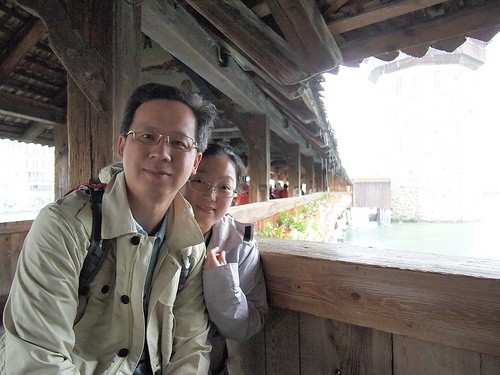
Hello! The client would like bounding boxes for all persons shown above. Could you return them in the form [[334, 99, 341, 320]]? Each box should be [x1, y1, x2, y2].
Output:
[[1, 81, 217, 375], [234, 178, 305, 206], [180, 140, 268, 375]]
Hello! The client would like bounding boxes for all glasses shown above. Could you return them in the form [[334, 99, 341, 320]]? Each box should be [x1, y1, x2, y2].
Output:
[[187, 178, 239, 198], [127, 128, 198, 151]]
